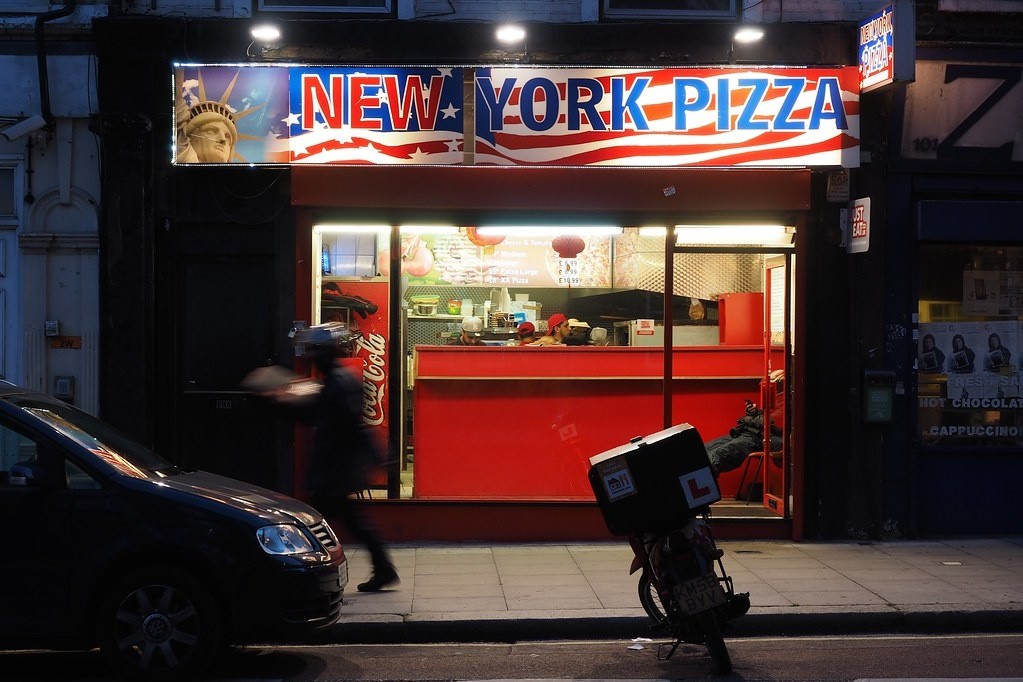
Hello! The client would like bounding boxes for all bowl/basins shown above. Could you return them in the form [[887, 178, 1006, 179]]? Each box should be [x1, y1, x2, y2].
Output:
[[410, 294, 440, 303]]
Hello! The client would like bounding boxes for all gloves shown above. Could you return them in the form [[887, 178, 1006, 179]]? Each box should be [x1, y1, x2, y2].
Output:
[[728, 416, 760, 438]]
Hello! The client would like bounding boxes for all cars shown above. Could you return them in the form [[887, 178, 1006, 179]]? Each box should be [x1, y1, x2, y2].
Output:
[[0, 375, 348, 682]]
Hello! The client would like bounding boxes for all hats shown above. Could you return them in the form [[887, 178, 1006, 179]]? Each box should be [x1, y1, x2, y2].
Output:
[[461, 316, 485, 337], [518, 322, 535, 336], [546, 314, 566, 336]]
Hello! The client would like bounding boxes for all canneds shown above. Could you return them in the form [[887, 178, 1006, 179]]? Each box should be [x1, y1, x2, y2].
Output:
[[447, 299, 461, 315]]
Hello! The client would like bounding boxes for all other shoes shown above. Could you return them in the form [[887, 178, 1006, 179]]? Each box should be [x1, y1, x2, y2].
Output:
[[357, 568, 400, 592]]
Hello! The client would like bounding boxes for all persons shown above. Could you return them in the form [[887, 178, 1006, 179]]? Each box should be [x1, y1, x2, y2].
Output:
[[252, 322, 397, 590], [918, 334, 945, 374], [977, 282, 987, 300], [961, 388, 969, 398], [705, 370, 784, 473], [983, 333, 1011, 372], [445, 316, 487, 346], [997, 387, 1004, 398], [518, 321, 536, 346], [948, 333, 976, 373], [532, 313, 571, 345]]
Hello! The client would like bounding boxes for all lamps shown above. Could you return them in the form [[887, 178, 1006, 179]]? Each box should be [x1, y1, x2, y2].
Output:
[[244, 20, 289, 62], [722, 23, 766, 65], [493, 23, 533, 68]]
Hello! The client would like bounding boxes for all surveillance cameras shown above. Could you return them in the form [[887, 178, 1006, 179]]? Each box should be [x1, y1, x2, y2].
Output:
[[0, 115, 47, 142]]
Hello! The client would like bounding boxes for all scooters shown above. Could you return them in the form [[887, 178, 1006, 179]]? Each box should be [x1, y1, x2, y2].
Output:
[[626, 504, 751, 676]]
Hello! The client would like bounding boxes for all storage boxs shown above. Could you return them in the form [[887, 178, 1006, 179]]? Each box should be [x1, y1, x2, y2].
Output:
[[590, 425, 723, 541]]
